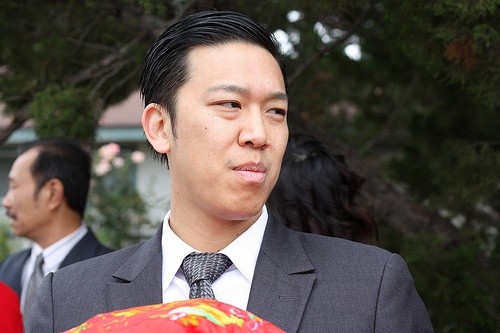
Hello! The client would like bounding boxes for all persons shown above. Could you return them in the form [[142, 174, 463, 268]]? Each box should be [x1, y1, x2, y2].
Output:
[[23, 11, 435, 333], [267, 133, 381, 247], [1, 140, 115, 298]]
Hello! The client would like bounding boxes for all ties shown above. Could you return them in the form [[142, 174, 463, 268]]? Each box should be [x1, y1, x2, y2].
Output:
[[180, 253, 233, 300], [22, 253, 45, 332]]
